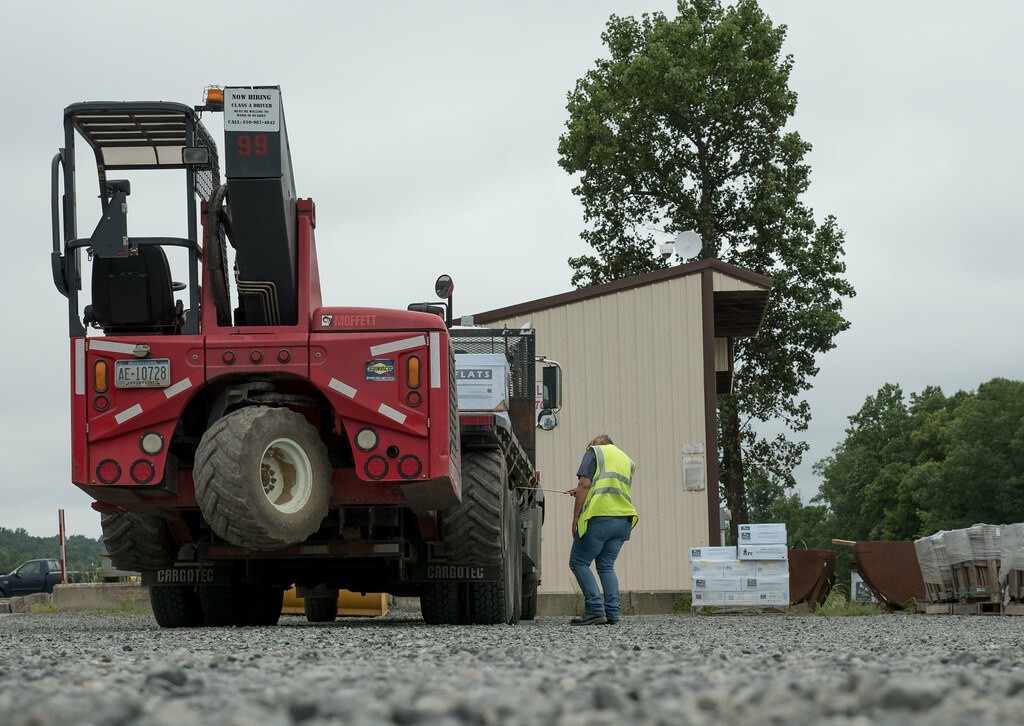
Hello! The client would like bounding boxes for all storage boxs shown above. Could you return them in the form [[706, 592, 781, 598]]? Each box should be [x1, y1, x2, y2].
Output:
[[692, 579, 741, 592], [691, 561, 723, 580], [692, 591, 725, 605], [755, 559, 789, 579], [689, 546, 737, 562], [738, 545, 788, 560], [725, 591, 758, 605], [723, 561, 756, 579], [742, 579, 789, 592], [739, 530, 787, 544], [738, 523, 785, 537], [758, 591, 790, 606]]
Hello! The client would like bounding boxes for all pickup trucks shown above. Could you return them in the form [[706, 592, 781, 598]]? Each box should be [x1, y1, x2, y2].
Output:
[[0, 557, 98, 599]]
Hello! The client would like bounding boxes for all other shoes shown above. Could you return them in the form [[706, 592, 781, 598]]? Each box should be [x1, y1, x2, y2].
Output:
[[595, 616, 619, 624], [571, 613, 606, 625]]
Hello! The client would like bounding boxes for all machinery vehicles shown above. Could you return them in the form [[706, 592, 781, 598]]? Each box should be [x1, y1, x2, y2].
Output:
[[50, 81, 563, 636]]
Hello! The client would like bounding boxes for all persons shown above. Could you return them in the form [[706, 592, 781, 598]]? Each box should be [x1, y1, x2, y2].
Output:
[[564, 435, 639, 627]]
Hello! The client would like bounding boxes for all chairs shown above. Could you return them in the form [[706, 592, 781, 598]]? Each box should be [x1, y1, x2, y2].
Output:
[[84, 243, 184, 335]]
